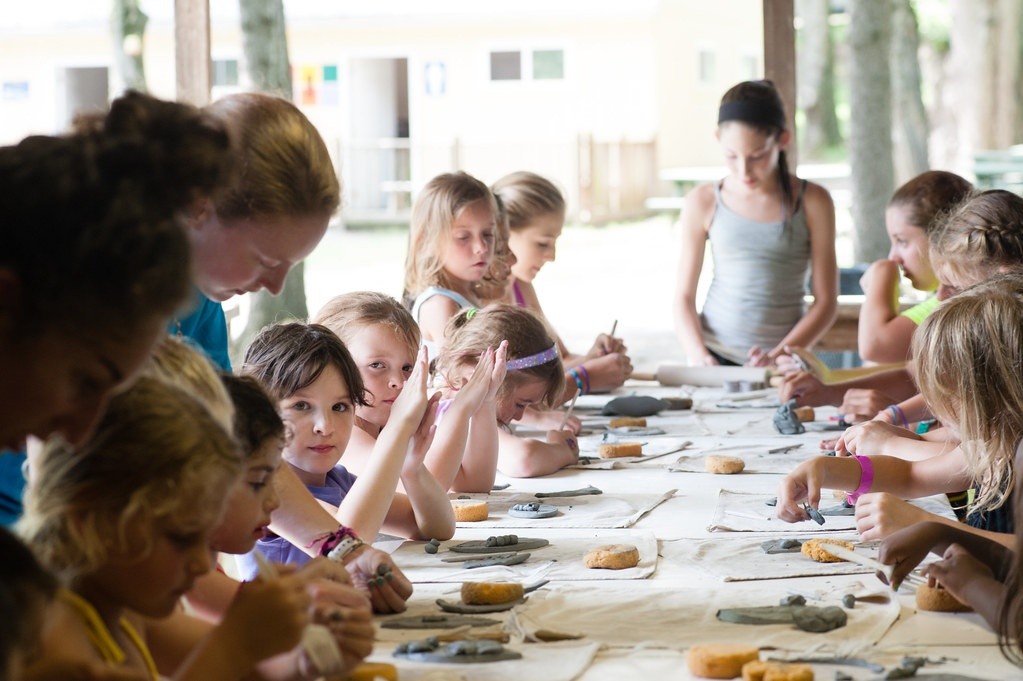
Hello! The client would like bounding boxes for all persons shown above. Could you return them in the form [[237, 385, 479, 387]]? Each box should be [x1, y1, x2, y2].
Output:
[[668, 77, 840, 368], [0, 84, 630, 681], [773, 167, 1023, 672]]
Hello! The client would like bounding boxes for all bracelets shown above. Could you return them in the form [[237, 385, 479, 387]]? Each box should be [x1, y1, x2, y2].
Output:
[[885, 405, 897, 425], [893, 403, 910, 429], [579, 365, 590, 394], [326, 538, 363, 564], [569, 367, 583, 397], [844, 454, 875, 506], [306, 527, 357, 556]]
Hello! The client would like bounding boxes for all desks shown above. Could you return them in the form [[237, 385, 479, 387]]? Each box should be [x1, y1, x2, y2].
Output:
[[286, 380, 1022, 681]]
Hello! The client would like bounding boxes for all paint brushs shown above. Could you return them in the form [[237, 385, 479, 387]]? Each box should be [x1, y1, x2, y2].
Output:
[[611, 320, 620, 335], [559, 386, 583, 430]]
